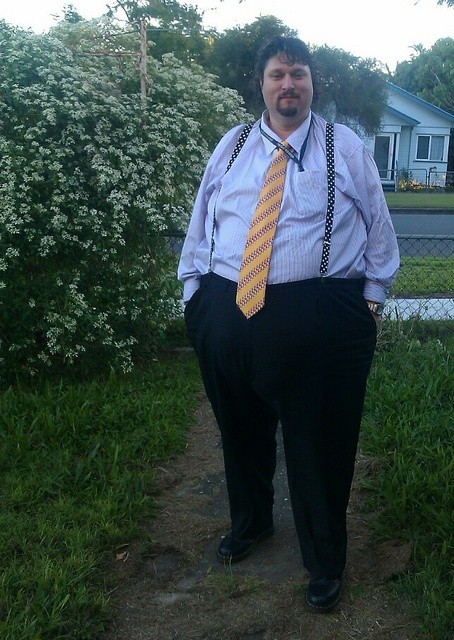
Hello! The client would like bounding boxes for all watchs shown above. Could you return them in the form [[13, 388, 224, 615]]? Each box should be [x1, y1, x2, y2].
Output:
[[366, 301, 383, 315]]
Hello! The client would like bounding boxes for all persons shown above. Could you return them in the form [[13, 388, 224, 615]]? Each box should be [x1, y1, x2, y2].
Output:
[[177, 36, 399, 614]]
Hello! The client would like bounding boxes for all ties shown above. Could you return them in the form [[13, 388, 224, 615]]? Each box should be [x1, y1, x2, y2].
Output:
[[236, 140, 290, 319]]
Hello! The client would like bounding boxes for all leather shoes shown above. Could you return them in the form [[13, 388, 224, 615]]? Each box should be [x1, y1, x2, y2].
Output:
[[216, 524, 274, 564], [305, 574, 344, 614]]
